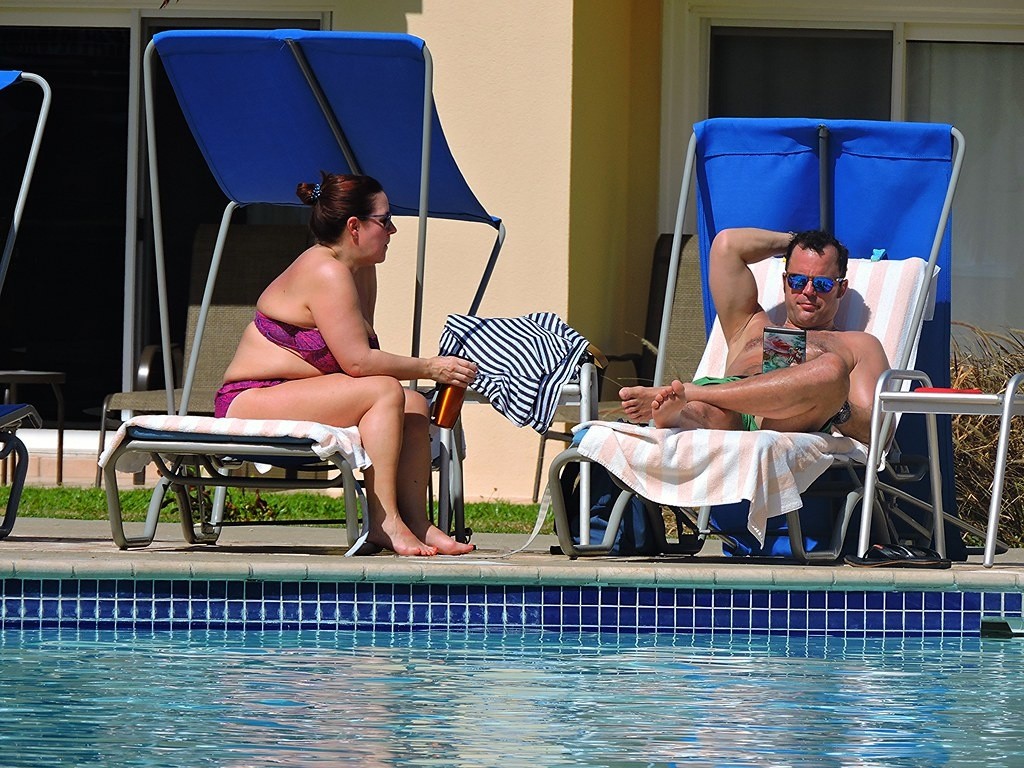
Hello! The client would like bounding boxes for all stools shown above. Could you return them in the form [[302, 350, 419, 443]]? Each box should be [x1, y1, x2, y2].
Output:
[[855, 368, 1023, 570], [0, 370, 67, 486], [419, 362, 602, 548]]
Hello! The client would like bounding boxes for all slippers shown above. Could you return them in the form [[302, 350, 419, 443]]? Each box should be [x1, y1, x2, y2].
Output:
[[887, 542, 951, 570], [845, 545, 942, 569]]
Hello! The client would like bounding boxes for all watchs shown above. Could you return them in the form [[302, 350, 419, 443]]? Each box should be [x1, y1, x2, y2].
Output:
[[830, 400, 851, 425]]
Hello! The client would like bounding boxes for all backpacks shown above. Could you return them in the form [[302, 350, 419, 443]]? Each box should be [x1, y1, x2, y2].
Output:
[[558, 418, 667, 555]]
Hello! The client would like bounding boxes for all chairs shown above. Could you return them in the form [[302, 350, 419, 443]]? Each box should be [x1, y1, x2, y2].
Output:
[[110, 303, 260, 490], [0, 401, 44, 540], [549, 260, 943, 562], [97, 387, 435, 555]]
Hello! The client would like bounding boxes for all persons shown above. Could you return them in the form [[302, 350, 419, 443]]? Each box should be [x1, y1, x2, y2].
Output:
[[213, 170, 478, 556], [619, 227, 896, 447]]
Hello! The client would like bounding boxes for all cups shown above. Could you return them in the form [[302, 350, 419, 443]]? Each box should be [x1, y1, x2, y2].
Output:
[[429, 353, 476, 429]]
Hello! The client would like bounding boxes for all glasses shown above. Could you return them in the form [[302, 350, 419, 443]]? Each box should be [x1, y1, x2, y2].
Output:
[[365, 213, 393, 230], [785, 273, 843, 293]]
